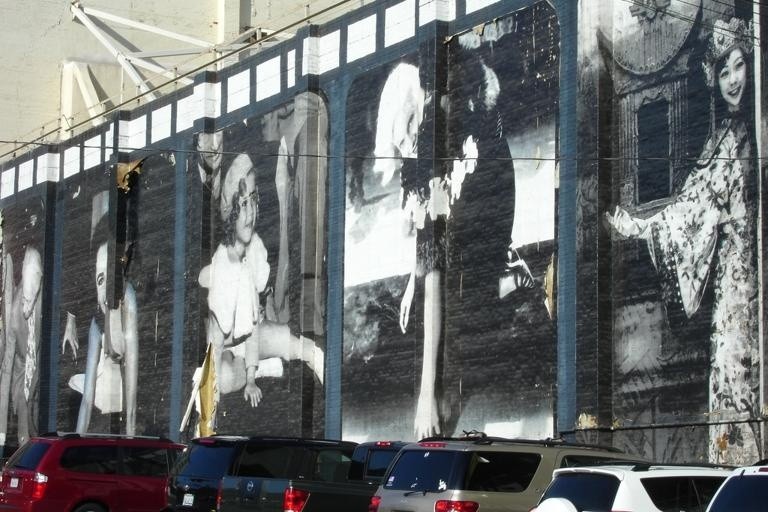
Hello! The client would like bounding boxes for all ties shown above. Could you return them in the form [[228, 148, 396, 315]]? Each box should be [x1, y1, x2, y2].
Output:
[[25, 314, 37, 393]]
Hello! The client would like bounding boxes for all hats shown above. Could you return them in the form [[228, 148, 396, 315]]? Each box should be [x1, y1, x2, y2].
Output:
[[219, 151, 255, 223]]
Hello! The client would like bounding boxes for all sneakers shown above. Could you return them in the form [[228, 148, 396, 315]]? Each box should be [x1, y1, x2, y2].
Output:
[[502, 245, 536, 291]]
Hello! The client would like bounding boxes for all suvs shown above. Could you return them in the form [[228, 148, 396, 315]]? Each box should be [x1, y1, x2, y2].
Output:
[[703, 458, 768, 512], [164, 434, 361, 512], [527, 460, 742, 512], [0, 430, 188, 512], [367, 430, 632, 512]]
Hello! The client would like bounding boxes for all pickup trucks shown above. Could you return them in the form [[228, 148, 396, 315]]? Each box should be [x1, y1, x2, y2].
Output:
[[214, 440, 411, 512]]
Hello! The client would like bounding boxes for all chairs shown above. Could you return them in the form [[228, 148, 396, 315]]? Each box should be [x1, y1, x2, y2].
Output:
[[316, 450, 343, 482]]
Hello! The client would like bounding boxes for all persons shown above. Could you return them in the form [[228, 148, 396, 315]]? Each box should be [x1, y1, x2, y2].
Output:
[[2, 245, 44, 448], [185, 109, 325, 434], [53, 209, 139, 440], [372, 63, 556, 438], [604, 17, 760, 465]]
